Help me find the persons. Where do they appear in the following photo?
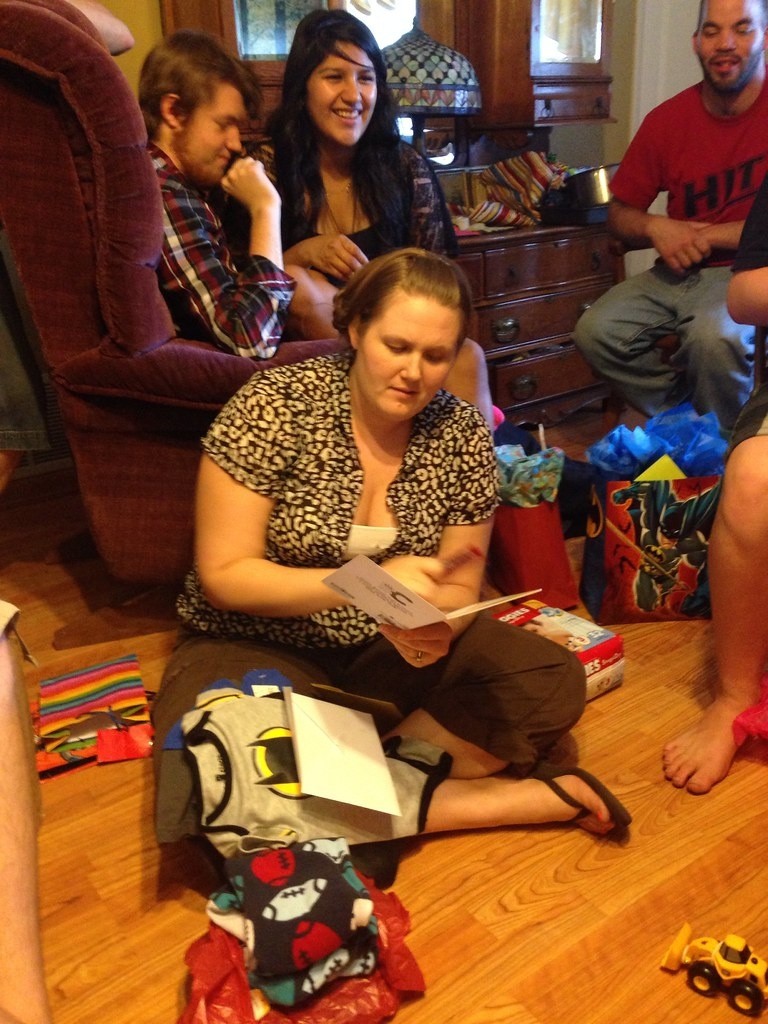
[150,246,632,879]
[661,168,767,795]
[136,6,507,445]
[570,0,768,433]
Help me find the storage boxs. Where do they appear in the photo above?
[492,598,627,700]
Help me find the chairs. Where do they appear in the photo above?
[0,0,349,585]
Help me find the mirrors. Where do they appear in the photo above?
[539,0,604,64]
[234,0,421,63]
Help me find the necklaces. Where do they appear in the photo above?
[325,194,357,235]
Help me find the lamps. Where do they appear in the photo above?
[380,13,483,158]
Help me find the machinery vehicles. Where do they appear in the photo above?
[659,921,768,1018]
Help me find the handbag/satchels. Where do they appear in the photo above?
[40,655,150,752]
[31,690,157,773]
[579,469,724,627]
[488,495,577,610]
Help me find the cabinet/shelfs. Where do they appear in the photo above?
[457,222,626,429]
[419,0,617,125]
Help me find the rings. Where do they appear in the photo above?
[416,651,423,661]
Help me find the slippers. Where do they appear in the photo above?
[506,763,632,832]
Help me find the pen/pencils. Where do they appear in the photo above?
[438,541,481,574]
